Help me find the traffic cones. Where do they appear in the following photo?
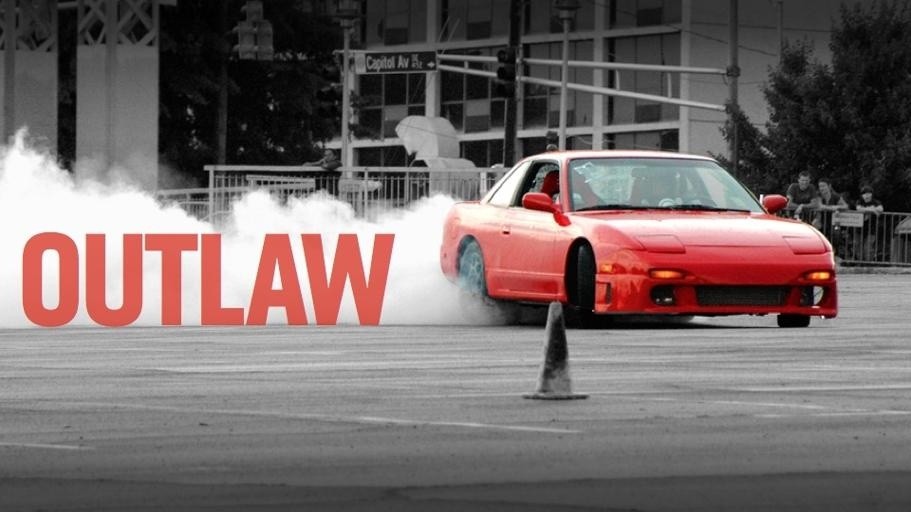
[515,294,594,401]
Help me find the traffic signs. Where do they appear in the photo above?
[349,49,440,77]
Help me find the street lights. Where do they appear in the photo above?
[554,0,583,151]
[334,0,364,208]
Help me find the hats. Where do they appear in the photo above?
[860,186,873,193]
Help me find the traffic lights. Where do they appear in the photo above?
[494,46,516,99]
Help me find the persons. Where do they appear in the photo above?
[855,185,883,267]
[787,170,817,225]
[642,171,691,208]
[816,177,849,257]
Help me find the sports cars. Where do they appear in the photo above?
[437,146,839,329]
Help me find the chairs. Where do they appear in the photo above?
[541,169,650,209]
